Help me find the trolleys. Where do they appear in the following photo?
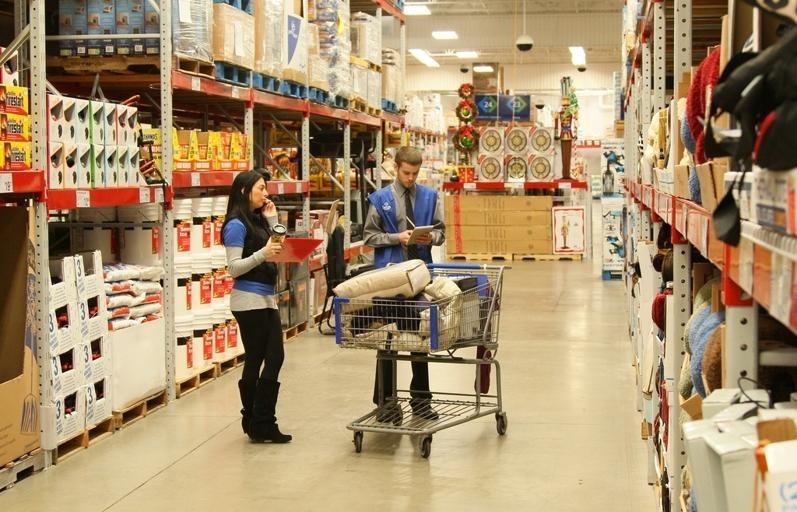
[330,264,512,460]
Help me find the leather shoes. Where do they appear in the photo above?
[377,413,392,423]
[409,401,438,420]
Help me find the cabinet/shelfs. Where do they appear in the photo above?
[0,0,402,487]
[598,1,794,512]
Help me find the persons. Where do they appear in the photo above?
[220,171,292,444]
[363,146,446,422]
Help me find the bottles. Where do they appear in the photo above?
[270,223,287,256]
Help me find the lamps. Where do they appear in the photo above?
[514,0,534,52]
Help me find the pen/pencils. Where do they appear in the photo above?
[404,215,417,228]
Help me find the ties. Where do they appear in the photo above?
[404,189,419,259]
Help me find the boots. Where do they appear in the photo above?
[238,379,292,443]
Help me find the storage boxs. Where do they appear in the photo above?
[442,191,555,258]
